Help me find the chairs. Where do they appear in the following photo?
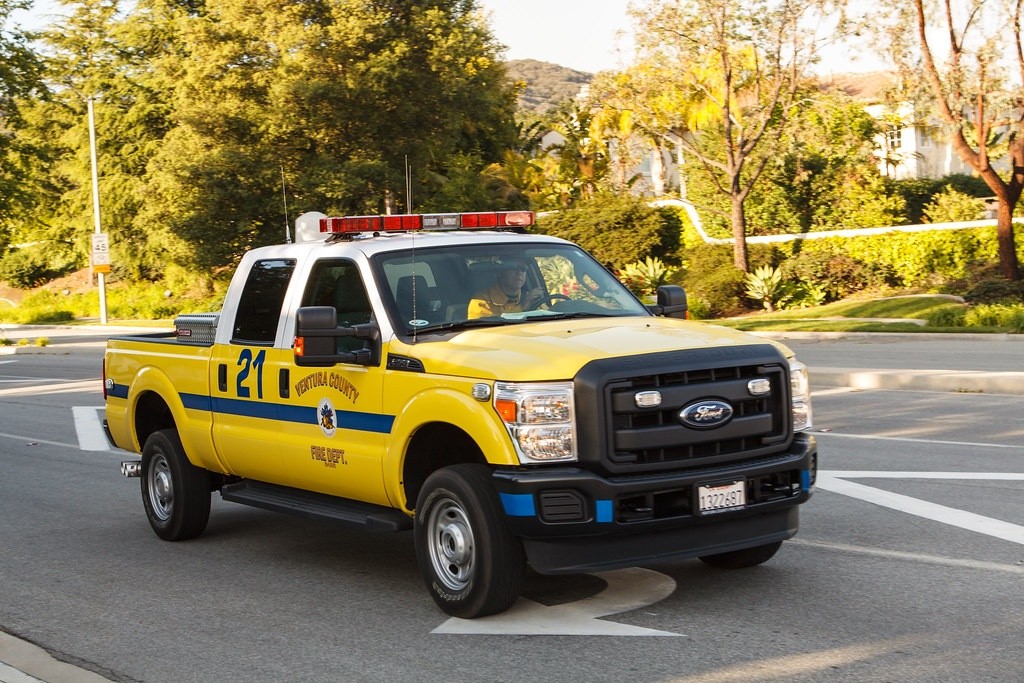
[331,274,438,329]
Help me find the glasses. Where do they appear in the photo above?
[508,263,529,271]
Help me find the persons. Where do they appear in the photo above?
[467,256,549,320]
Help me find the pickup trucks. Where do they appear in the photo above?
[96,209,822,621]
